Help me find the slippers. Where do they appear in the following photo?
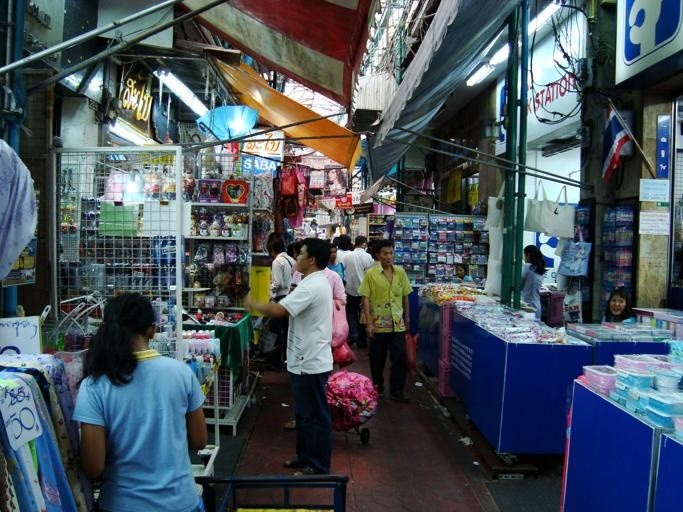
[294,464,329,475]
[285,456,313,468]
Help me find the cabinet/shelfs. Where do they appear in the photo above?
[568,325,673,367]
[181,179,253,310]
[563,379,674,512]
[653,433,683,512]
[239,177,271,344]
[451,300,592,454]
[182,312,254,410]
[367,213,394,243]
[417,294,443,379]
[394,212,428,287]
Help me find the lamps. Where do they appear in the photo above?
[154,70,206,117]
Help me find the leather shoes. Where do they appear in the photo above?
[390,395,410,403]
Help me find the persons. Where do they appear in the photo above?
[327,244,346,281]
[72,292,208,512]
[242,237,333,475]
[335,234,352,287]
[357,239,414,403]
[521,245,549,319]
[342,235,375,347]
[450,264,472,283]
[284,268,347,430]
[266,234,298,361]
[601,289,636,323]
